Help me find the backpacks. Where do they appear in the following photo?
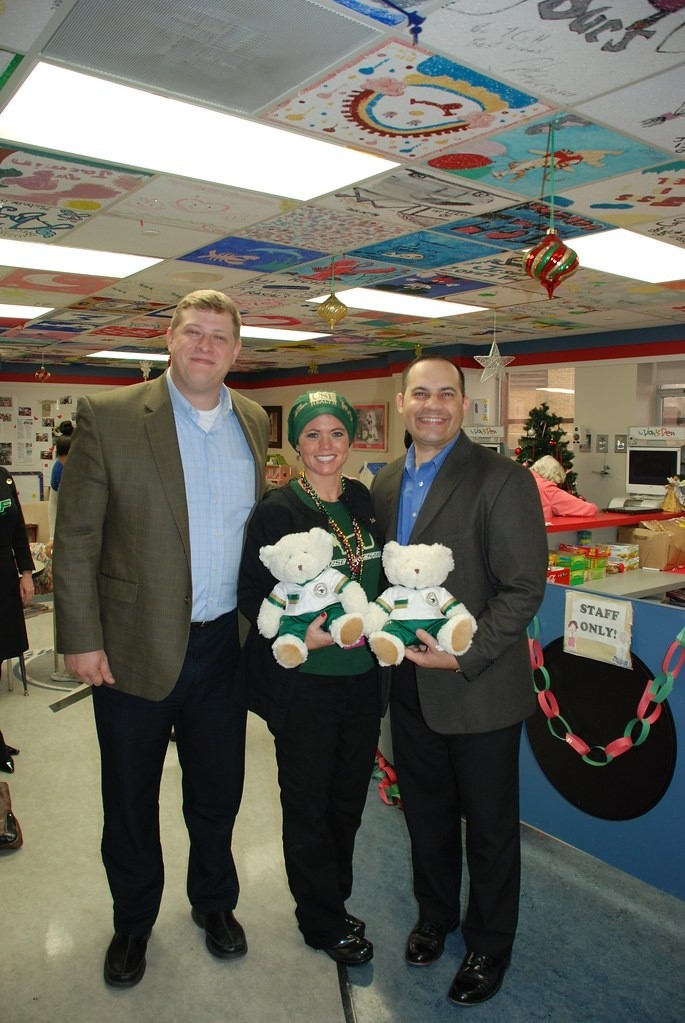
[0,782,22,851]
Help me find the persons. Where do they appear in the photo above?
[50,421,86,685]
[237,389,391,967]
[0,465,38,774]
[52,289,277,987]
[369,354,549,1007]
[529,455,599,519]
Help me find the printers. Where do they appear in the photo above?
[609,497,665,510]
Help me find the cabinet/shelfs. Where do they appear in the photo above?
[265,464,292,488]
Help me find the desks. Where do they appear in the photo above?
[14,558,47,696]
[543,511,685,551]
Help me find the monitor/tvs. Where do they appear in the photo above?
[479,444,500,453]
[626,447,681,495]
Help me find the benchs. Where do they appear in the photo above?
[570,567,685,599]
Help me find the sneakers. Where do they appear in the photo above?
[50,670,83,682]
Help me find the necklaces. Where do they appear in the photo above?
[300,469,364,584]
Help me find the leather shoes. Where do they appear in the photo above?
[449,951,512,1004]
[103,928,154,987]
[0,745,20,774]
[191,906,248,960]
[406,917,460,964]
[322,914,374,966]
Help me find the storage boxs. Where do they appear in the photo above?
[546,517,685,586]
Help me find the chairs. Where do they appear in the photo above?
[8,543,52,693]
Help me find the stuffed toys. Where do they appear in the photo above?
[257,527,373,668]
[365,540,478,667]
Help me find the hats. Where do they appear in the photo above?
[287,390,359,449]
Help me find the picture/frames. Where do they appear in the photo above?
[350,402,388,453]
[261,406,282,449]
